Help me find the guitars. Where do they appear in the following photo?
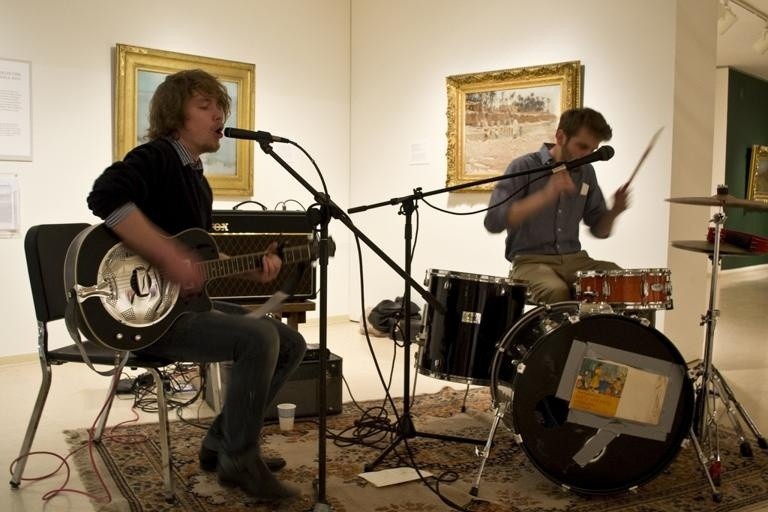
[65,221,334,351]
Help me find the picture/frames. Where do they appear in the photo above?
[443,59,582,195]
[112,42,256,200]
[745,144,768,204]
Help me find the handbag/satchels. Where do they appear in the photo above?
[368,297,421,332]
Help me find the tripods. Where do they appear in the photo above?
[347,164,555,470]
[689,211,768,502]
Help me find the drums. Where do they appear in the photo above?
[415,269,531,387]
[492,300,695,496]
[576,269,673,310]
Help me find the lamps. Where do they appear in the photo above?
[717,1,768,56]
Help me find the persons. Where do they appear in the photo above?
[478,117,522,139]
[85,65,310,501]
[477,101,630,311]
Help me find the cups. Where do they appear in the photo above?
[277,403,297,433]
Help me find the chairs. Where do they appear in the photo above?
[8,221,222,503]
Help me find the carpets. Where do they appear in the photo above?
[60,386,766,512]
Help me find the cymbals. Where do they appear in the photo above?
[672,241,765,255]
[664,196,766,210]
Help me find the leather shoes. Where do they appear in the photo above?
[217,467,300,499]
[199,444,286,472]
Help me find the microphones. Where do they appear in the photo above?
[224,127,289,143]
[256,131,448,512]
[552,145,614,174]
